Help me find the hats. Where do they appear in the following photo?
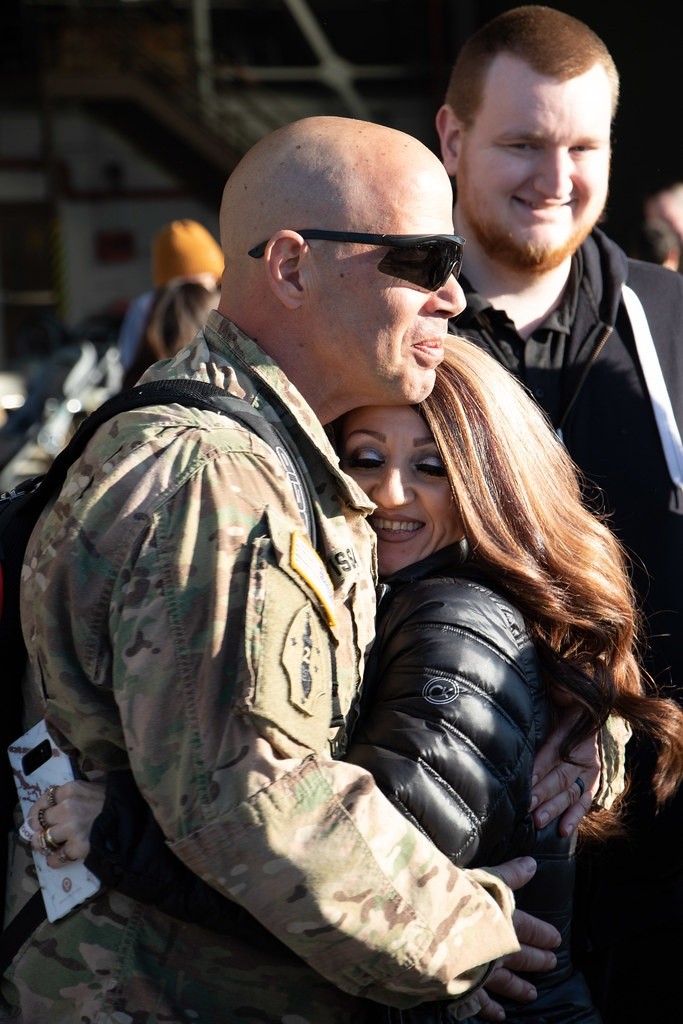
[150,218,226,287]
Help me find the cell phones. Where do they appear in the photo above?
[8,720,105,924]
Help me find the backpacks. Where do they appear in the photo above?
[0,379,319,973]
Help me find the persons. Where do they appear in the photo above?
[0,4,683,1024]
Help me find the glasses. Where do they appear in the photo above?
[248,228,466,292]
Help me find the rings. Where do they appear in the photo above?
[19,817,35,840]
[38,809,48,828]
[47,785,58,805]
[60,847,78,863]
[576,778,585,797]
[38,827,59,854]
